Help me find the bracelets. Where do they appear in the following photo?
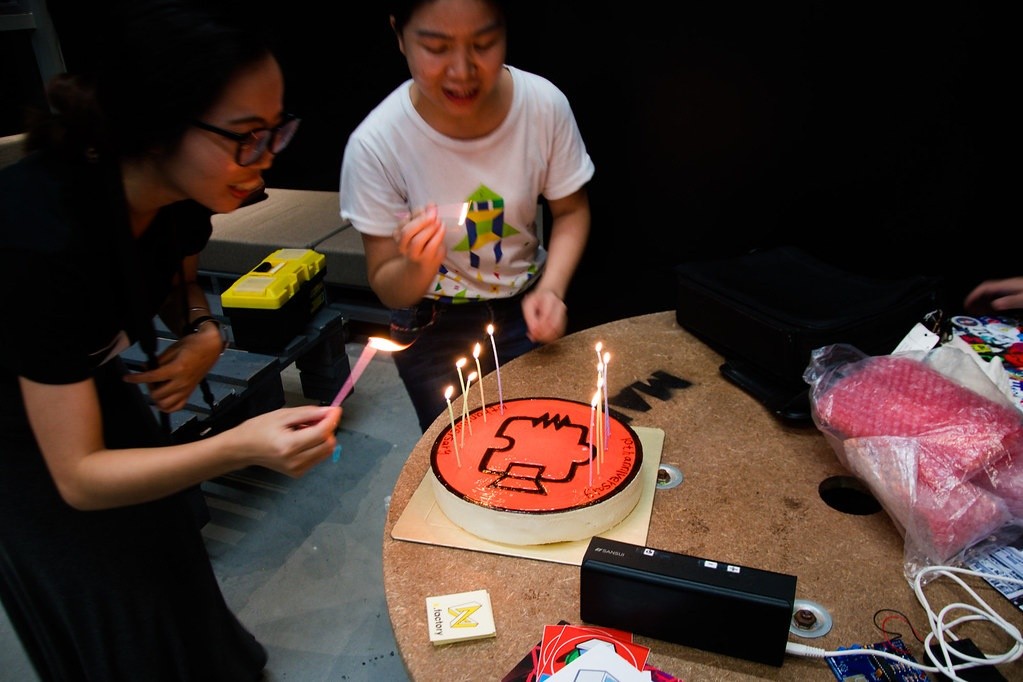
[188,305,211,315]
[191,315,230,356]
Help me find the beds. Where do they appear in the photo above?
[198,187,393,326]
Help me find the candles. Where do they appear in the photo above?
[444,386,462,469]
[330,336,417,406]
[487,323,503,415]
[473,341,486,422]
[589,341,610,486]
[460,372,477,448]
[456,357,472,436]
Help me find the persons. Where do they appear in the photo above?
[964,273,1023,311]
[0,12,343,681]
[340,2,596,447]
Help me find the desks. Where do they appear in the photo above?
[382,309,1023,682]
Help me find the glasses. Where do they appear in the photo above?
[193,111,301,167]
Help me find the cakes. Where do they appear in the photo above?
[428,396,646,547]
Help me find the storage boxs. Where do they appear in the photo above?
[221,249,327,354]
